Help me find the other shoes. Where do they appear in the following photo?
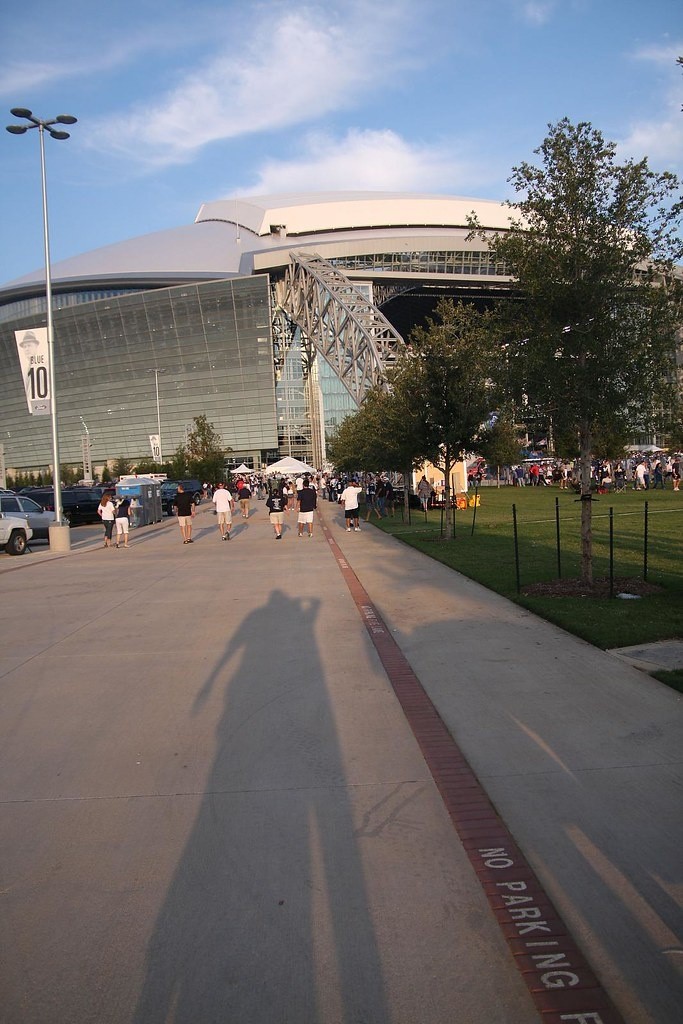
[560,486,564,490]
[564,487,568,489]
[350,523,359,527]
[124,545,129,548]
[117,542,120,548]
[345,528,350,532]
[354,527,361,531]
[653,487,656,489]
[222,532,230,541]
[662,487,666,489]
[288,508,294,510]
[673,486,680,491]
[637,487,641,491]
[308,533,313,537]
[275,535,281,539]
[183,539,192,544]
[299,533,302,537]
[104,542,107,548]
[242,514,248,518]
[645,487,649,490]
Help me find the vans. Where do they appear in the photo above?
[467,457,487,479]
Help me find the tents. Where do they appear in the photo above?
[261,457,318,475]
[230,463,255,474]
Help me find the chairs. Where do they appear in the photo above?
[569,481,629,496]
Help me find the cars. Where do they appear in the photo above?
[0,513,34,556]
[0,495,70,539]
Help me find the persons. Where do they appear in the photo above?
[201,472,321,501]
[496,447,683,493]
[97,493,119,548]
[212,482,235,541]
[265,489,290,539]
[468,469,482,490]
[173,485,195,544]
[114,497,134,548]
[237,483,252,519]
[296,479,318,537]
[316,468,395,532]
[418,476,433,512]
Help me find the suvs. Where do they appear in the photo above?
[0,478,205,524]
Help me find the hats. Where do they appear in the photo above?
[219,483,223,486]
[383,477,389,480]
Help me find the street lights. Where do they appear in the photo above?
[146,366,168,465]
[5,107,78,556]
